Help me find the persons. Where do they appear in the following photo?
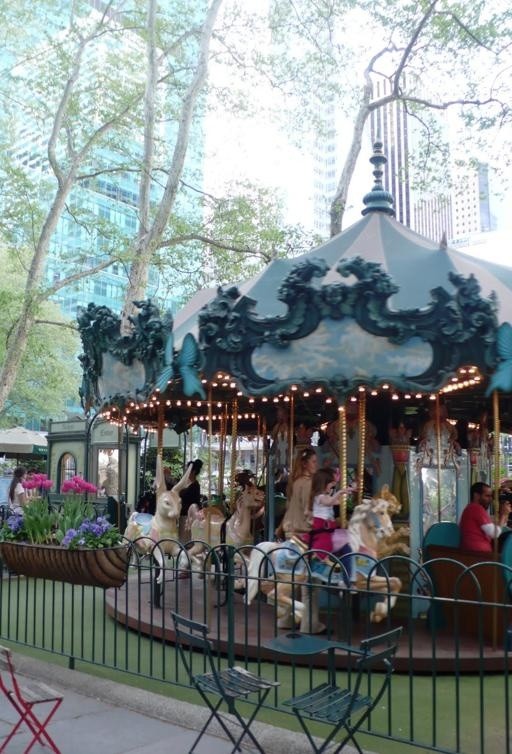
[8,466,28,576]
[458,482,512,553]
[165,459,203,579]
[277,447,327,634]
[301,466,358,595]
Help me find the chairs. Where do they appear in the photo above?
[168,608,404,754]
[1,642,61,753]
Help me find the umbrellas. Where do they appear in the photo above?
[0,426,48,467]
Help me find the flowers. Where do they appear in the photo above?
[1,473,126,548]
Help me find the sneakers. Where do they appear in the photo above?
[175,572,189,579]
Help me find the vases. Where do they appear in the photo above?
[3,542,130,590]
